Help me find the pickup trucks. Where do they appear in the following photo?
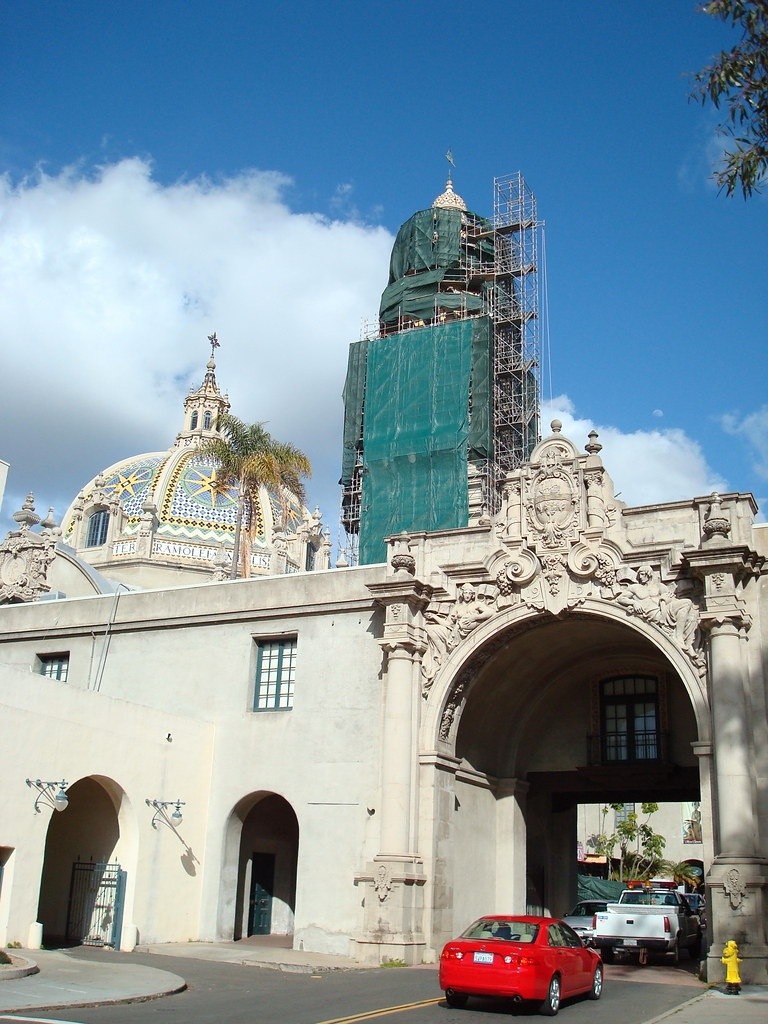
[593,880,707,965]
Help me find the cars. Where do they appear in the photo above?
[439,914,604,1017]
[560,900,625,959]
[680,890,706,925]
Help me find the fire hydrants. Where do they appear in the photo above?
[720,939,744,995]
